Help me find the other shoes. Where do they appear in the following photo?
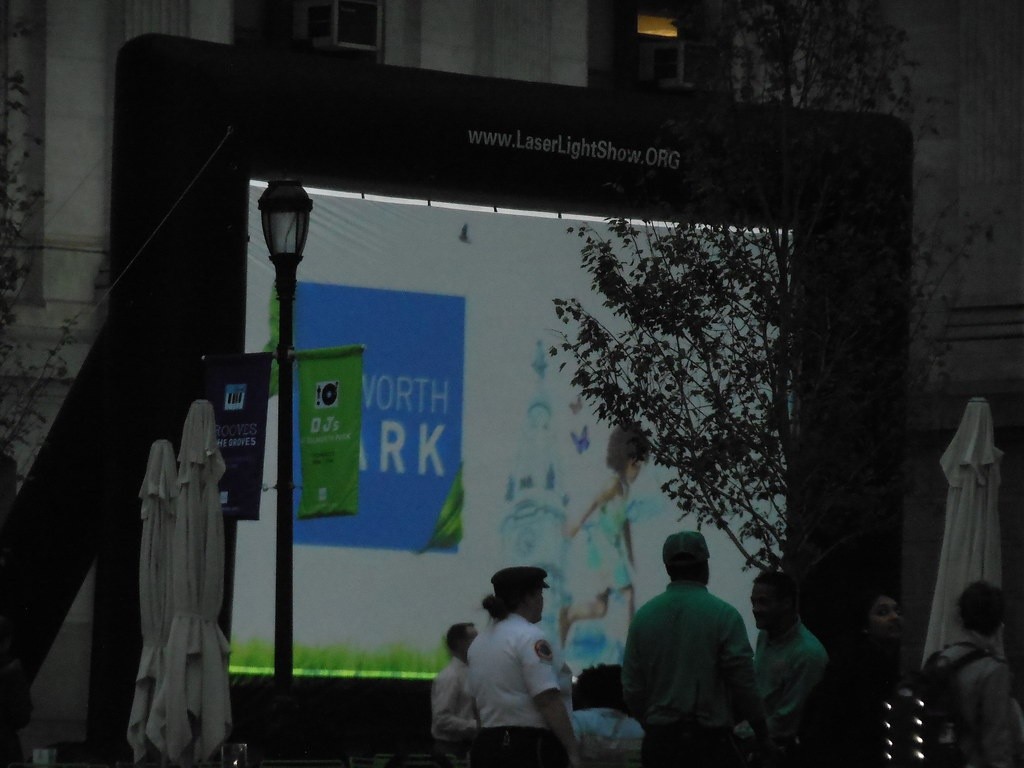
[559,607,572,650]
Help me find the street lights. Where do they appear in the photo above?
[257,174,314,762]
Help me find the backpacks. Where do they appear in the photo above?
[884,642,994,768]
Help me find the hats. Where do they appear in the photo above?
[662,530,710,566]
[491,567,551,596]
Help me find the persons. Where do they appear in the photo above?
[558,420,650,649]
[429,531,1024,768]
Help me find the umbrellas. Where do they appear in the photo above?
[920,396,1006,666]
[126,398,233,768]
